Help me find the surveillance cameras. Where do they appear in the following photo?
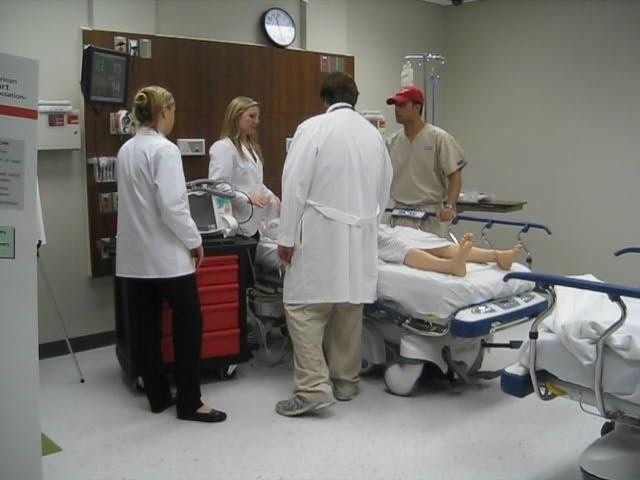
[452,0,462,6]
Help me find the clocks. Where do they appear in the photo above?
[261,6,296,47]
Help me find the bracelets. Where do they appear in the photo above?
[444,204,455,209]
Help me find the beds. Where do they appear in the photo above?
[254,205,553,397]
[501,246,639,479]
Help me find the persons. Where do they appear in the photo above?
[208,97,280,352]
[264,217,524,278]
[113,86,227,423]
[382,83,467,238]
[274,72,393,416]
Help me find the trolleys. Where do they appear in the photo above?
[115,232,257,392]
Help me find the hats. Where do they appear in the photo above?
[387,85,424,105]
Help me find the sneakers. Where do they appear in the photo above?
[151,392,226,422]
[276,386,359,416]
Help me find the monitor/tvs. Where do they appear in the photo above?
[80,45,130,107]
